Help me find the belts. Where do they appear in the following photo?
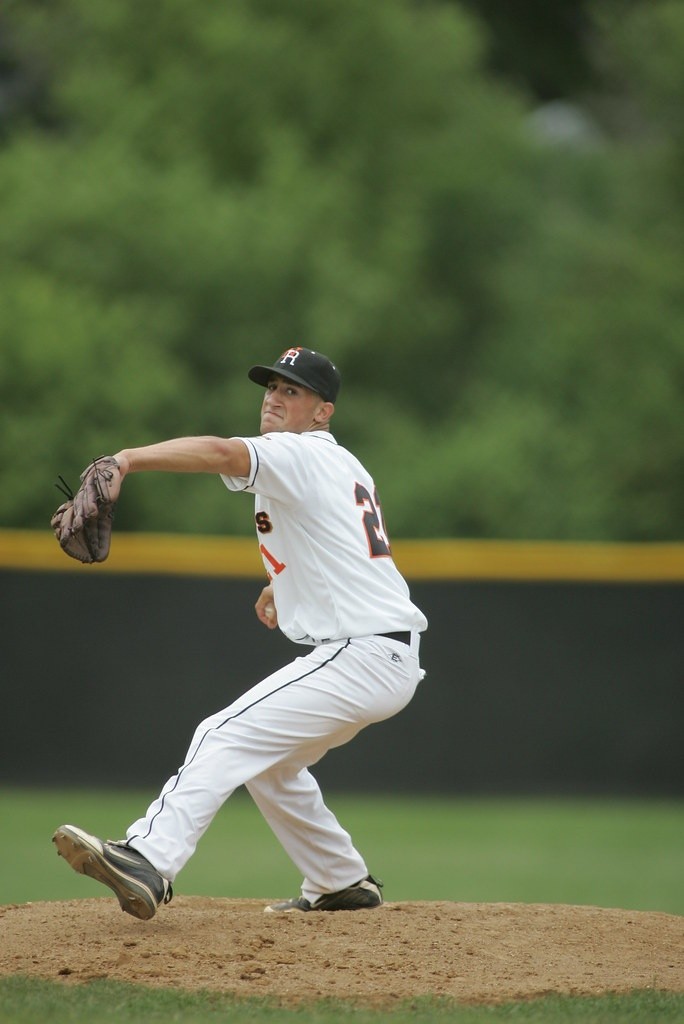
[377,631,414,645]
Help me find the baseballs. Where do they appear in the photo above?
[265,602,276,620]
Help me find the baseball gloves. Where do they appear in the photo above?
[48,454,122,562]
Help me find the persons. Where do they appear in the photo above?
[50,347,428,916]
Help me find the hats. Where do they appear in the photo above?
[248,347,340,406]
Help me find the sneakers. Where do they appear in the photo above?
[263,875,385,912]
[52,824,174,921]
